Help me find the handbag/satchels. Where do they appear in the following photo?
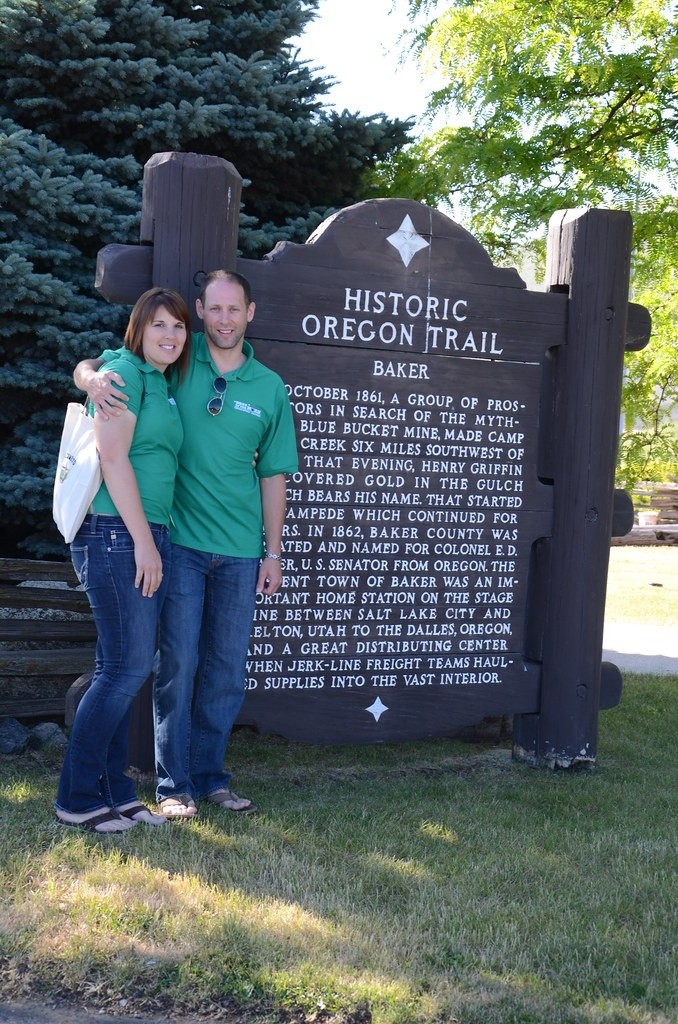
[53,360,148,544]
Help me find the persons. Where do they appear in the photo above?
[73,270,299,818]
[54,290,193,834]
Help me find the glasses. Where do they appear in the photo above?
[207,375,228,416]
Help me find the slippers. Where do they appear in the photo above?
[54,808,136,835]
[158,794,200,818]
[206,790,257,815]
[119,804,171,827]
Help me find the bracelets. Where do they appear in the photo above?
[265,553,282,561]
